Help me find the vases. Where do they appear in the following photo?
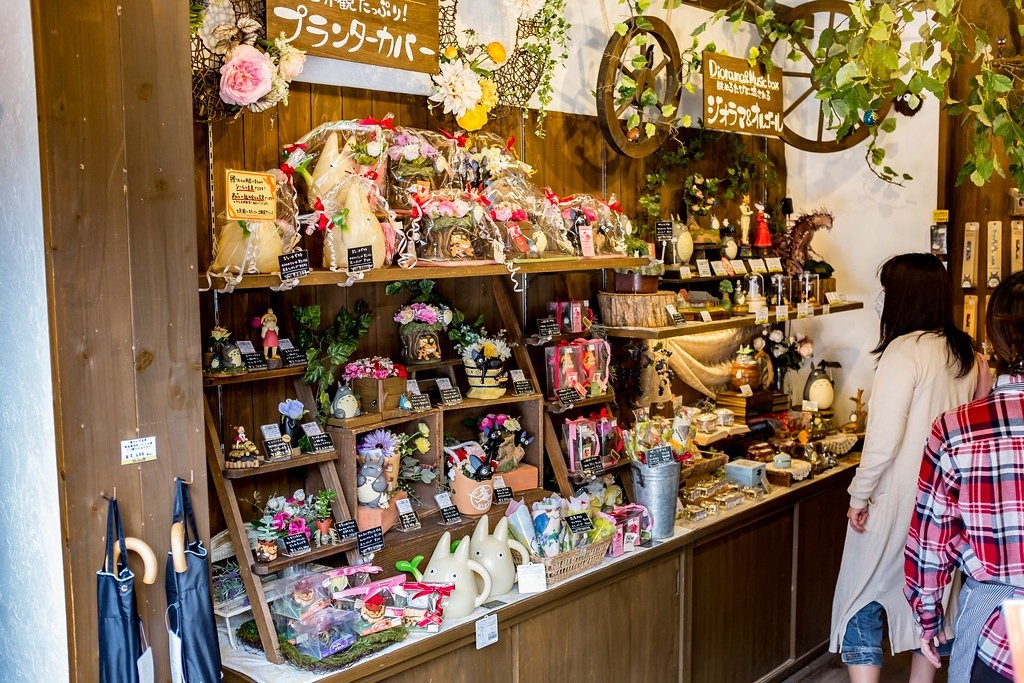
[463,359,510,400]
[479,431,526,472]
[399,320,444,365]
[279,413,304,449]
[257,539,278,562]
[730,363,762,392]
[355,449,401,492]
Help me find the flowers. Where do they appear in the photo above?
[339,353,396,381]
[359,423,433,461]
[278,396,305,426]
[504,491,620,558]
[410,193,475,229]
[214,16,306,116]
[388,131,444,177]
[450,323,513,367]
[575,422,594,439]
[209,558,252,607]
[596,421,615,441]
[753,328,814,370]
[265,489,315,541]
[391,298,449,329]
[424,28,508,132]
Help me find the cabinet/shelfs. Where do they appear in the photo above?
[201,286,375,665]
[199,254,864,339]
[219,451,864,683]
[491,273,636,510]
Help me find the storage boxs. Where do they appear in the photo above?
[543,300,626,474]
[626,507,652,545]
[600,515,634,559]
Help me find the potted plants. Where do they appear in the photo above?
[315,489,337,536]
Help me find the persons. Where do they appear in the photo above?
[754,203,772,248]
[739,193,754,244]
[233,425,248,449]
[261,312,280,361]
[901,271,1024,683]
[827,252,980,683]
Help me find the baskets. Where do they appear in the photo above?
[513,526,615,587]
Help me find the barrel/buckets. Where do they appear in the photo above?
[629,461,682,539]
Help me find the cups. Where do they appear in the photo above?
[453,465,494,515]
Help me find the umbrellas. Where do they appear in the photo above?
[96,537,157,683]
[165,523,222,682]
